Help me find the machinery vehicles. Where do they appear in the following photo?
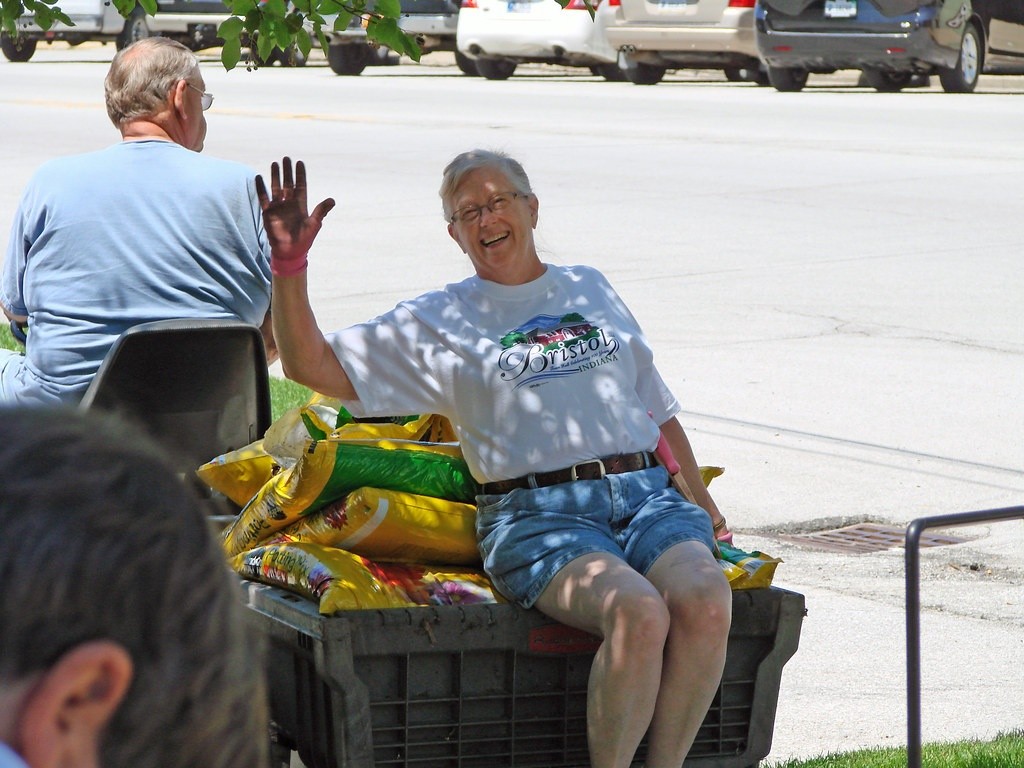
[0,320,809,767]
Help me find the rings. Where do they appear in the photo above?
[280,197,293,201]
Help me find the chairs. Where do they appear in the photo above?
[75,318,274,530]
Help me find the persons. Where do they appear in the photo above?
[256,148,734,768]
[0,398,275,768]
[0,35,285,423]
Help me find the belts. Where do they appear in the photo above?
[476,451,659,494]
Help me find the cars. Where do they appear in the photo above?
[286,1,482,79]
[597,0,770,87]
[0,1,151,65]
[754,0,1024,95]
[144,1,310,69]
[456,0,631,83]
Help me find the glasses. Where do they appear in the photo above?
[173,79,215,111]
[450,192,529,225]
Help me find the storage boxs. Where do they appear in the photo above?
[220,575,811,768]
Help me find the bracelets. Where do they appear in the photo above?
[269,252,309,276]
[714,517,726,531]
[716,532,733,542]
